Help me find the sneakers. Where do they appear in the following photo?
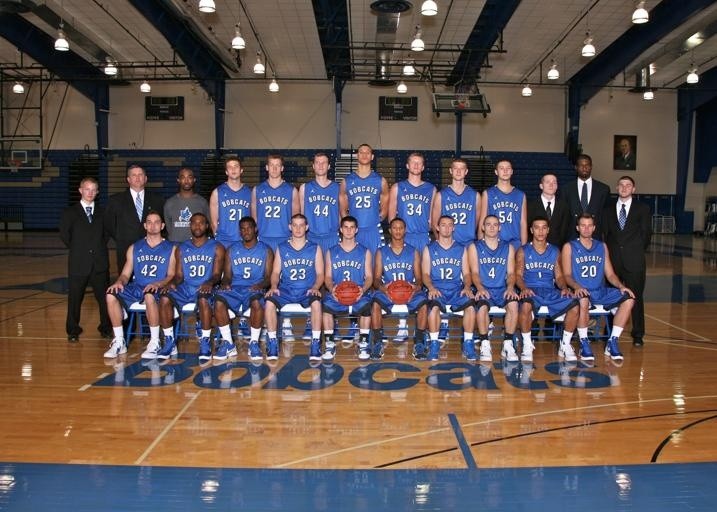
[103,337,127,357]
[604,336,624,360]
[213,361,233,371]
[267,361,278,373]
[141,337,160,358]
[502,358,518,376]
[248,361,262,375]
[341,322,359,343]
[557,339,577,361]
[158,359,174,372]
[198,337,212,360]
[195,320,202,339]
[428,340,440,361]
[393,325,409,341]
[283,342,295,358]
[282,324,295,342]
[519,361,536,376]
[309,338,322,361]
[412,343,428,360]
[577,358,594,368]
[500,339,518,361]
[604,356,623,369]
[322,335,336,359]
[439,322,450,342]
[520,339,535,361]
[265,337,278,360]
[557,359,575,375]
[462,339,480,360]
[358,360,368,381]
[141,359,161,371]
[104,354,126,370]
[310,361,323,382]
[199,360,212,377]
[213,340,238,360]
[301,320,312,340]
[237,319,250,339]
[248,340,263,361]
[334,320,340,340]
[156,335,179,359]
[322,361,335,384]
[578,337,595,360]
[370,342,385,360]
[356,334,370,359]
[394,341,408,358]
[479,340,492,362]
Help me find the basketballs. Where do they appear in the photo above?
[387,280,413,304]
[334,280,360,306]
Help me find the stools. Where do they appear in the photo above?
[215,303,261,354]
[529,305,566,353]
[432,302,474,353]
[377,300,420,351]
[329,305,363,353]
[275,302,324,355]
[579,303,614,353]
[123,300,163,347]
[481,304,518,353]
[174,302,204,351]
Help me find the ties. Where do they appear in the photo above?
[546,202,551,220]
[86,207,93,223]
[619,204,626,230]
[581,183,588,208]
[136,193,142,221]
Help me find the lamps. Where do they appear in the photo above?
[582,38,596,57]
[644,90,655,100]
[547,65,560,80]
[520,84,532,96]
[268,79,280,92]
[198,0,218,14]
[104,57,116,75]
[685,65,699,85]
[403,65,416,76]
[231,23,246,50]
[632,1,650,24]
[139,80,150,93]
[13,81,25,93]
[396,82,408,94]
[420,0,439,18]
[252,52,266,75]
[410,33,425,53]
[55,23,69,51]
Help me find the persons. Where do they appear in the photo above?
[299,153,341,340]
[214,216,275,360]
[480,160,528,343]
[323,216,374,360]
[112,164,160,277]
[388,153,437,342]
[164,168,209,340]
[468,215,520,362]
[266,213,324,360]
[210,157,252,341]
[158,213,225,360]
[561,155,612,341]
[104,211,176,359]
[372,218,428,360]
[339,144,390,344]
[421,216,478,362]
[252,154,300,342]
[617,140,635,168]
[517,218,579,361]
[562,215,635,361]
[431,158,482,346]
[528,173,571,343]
[60,179,115,343]
[605,176,652,347]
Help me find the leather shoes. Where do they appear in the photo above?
[633,337,643,346]
[68,334,78,341]
